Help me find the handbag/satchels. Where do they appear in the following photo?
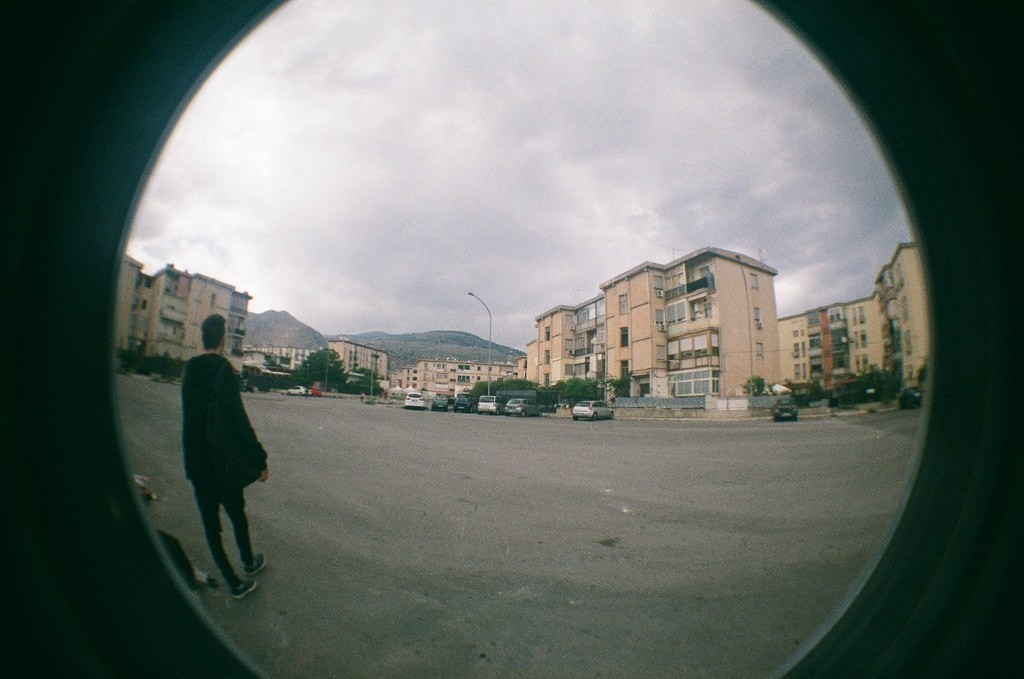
[223,440,266,489]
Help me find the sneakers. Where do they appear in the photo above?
[244,552,265,575]
[230,580,257,599]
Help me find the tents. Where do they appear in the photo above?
[386,385,418,407]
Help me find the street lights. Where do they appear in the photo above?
[468,292,493,396]
[734,254,756,418]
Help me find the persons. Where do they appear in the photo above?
[181,314,269,598]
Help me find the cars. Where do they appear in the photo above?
[504,398,542,417]
[404,392,424,408]
[771,396,798,421]
[900,386,923,410]
[476,395,507,415]
[286,384,313,396]
[572,400,614,421]
[453,397,474,413]
[430,395,450,411]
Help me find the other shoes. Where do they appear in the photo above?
[196,574,221,587]
